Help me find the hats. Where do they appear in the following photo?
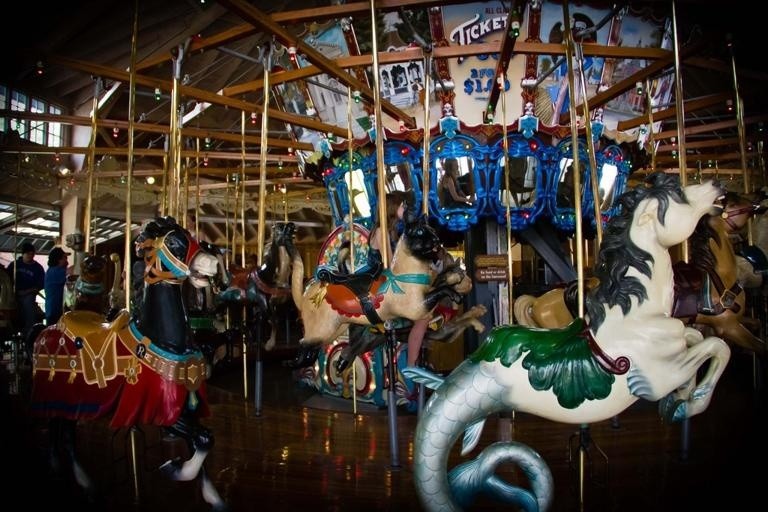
[48,247,72,260]
[21,242,36,254]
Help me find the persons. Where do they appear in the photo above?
[5,243,70,323]
